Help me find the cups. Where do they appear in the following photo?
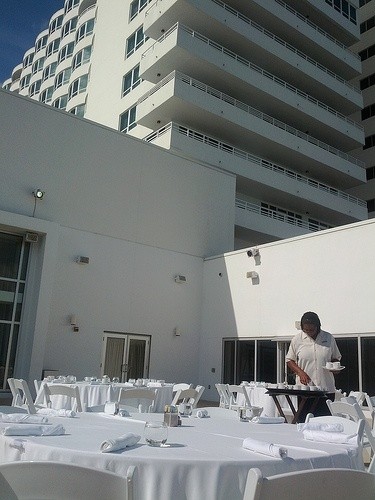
[144,420,168,447]
[242,380,272,387]
[326,361,341,369]
[268,382,322,391]
[84,375,120,384]
[128,378,165,387]
[239,407,249,420]
[47,375,77,383]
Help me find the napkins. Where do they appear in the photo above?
[119,409,129,417]
[297,422,344,432]
[251,417,284,424]
[196,409,208,419]
[101,432,139,452]
[39,407,76,418]
[303,430,359,445]
[2,412,48,424]
[242,437,288,459]
[3,424,64,436]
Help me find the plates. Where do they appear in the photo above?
[321,365,346,371]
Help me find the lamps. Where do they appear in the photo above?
[70,315,76,325]
[26,233,39,242]
[175,274,185,284]
[175,328,180,336]
[75,256,90,265]
[32,187,45,200]
[246,271,258,279]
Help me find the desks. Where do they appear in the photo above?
[40,378,174,412]
[265,387,335,425]
[0,412,365,500]
[236,386,297,417]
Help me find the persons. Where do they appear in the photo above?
[285,312,342,423]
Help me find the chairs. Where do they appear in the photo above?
[243,467,375,499]
[0,461,137,500]
[7,376,375,472]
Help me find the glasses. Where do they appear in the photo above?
[302,326,316,333]
